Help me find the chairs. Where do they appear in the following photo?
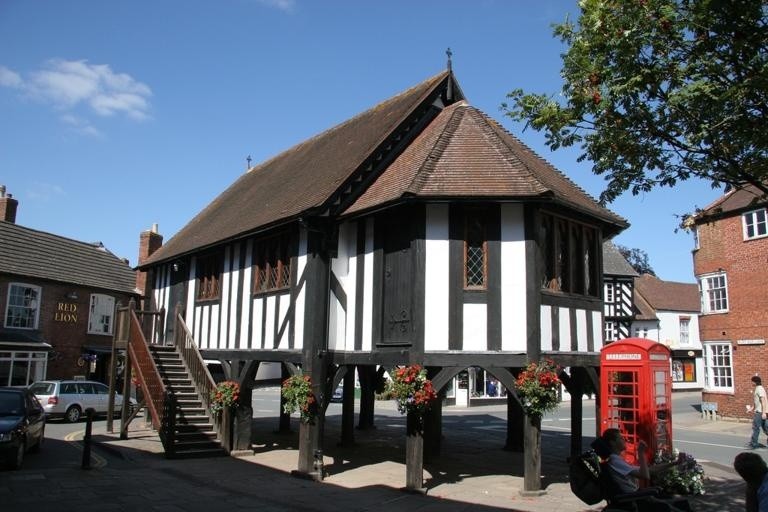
[576,448,680,512]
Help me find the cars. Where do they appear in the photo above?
[0,387,44,471]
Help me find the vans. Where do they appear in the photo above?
[24,378,142,423]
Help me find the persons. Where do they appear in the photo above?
[733,453,768,511]
[550,274,563,291]
[599,428,683,510]
[745,376,768,450]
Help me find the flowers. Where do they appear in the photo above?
[281,375,318,424]
[209,381,241,420]
[374,364,438,420]
[652,451,707,497]
[513,359,564,418]
